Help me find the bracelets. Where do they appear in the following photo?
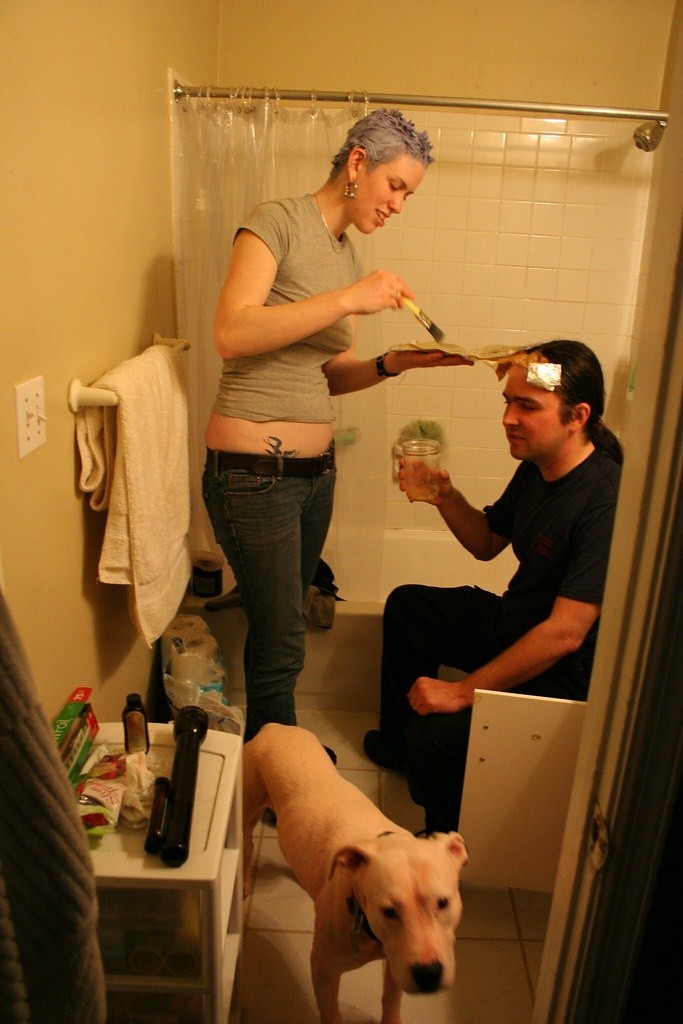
[375,353,402,377]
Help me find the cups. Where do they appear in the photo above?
[193,560,223,598]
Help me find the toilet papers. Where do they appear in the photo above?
[159,614,220,687]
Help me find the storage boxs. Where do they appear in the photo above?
[50,687,100,786]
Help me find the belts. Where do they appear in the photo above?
[203,441,335,478]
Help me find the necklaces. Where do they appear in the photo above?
[312,194,347,255]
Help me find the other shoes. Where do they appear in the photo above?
[365,730,397,771]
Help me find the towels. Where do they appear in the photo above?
[73,348,217,648]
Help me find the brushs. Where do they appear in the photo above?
[403,297,445,344]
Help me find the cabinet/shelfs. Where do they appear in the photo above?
[88,721,246,1024]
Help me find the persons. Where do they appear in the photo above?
[201,108,475,825]
[362,337,625,839]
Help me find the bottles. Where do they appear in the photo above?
[120,693,150,755]
[401,437,439,503]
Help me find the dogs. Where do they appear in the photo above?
[243,723,467,1024]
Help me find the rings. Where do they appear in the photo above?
[392,288,400,298]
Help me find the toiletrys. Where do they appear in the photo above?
[121,692,151,756]
